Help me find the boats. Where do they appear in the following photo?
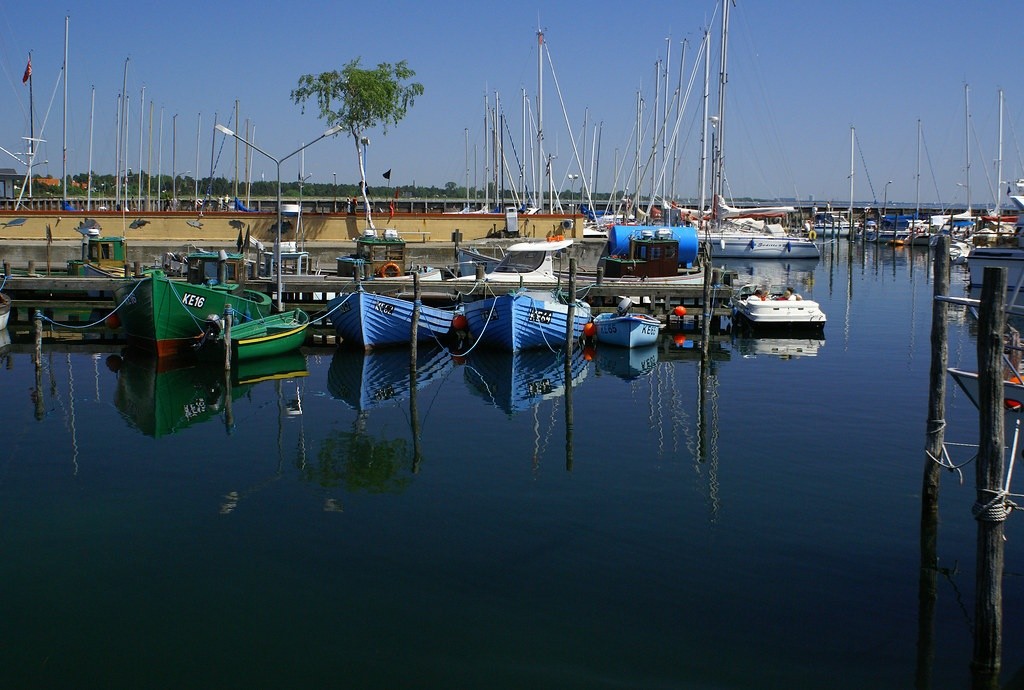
[932,269,1024,461]
[594,297,662,349]
[186,308,309,363]
[110,266,273,358]
[106,337,827,440]
[0,292,12,332]
[730,283,827,332]
[323,263,464,352]
[461,284,593,353]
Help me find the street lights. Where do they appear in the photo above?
[567,174,579,214]
[214,123,339,312]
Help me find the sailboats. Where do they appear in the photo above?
[0,16,1024,338]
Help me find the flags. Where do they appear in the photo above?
[1006,186,1011,196]
[23,56,32,84]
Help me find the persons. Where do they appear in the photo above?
[804,220,810,233]
[131,193,231,211]
[773,214,783,224]
[346,194,358,213]
[684,211,692,226]
[741,286,803,302]
[813,201,830,212]
[964,227,971,237]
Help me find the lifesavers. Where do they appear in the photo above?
[807,230,818,241]
[380,263,401,278]
